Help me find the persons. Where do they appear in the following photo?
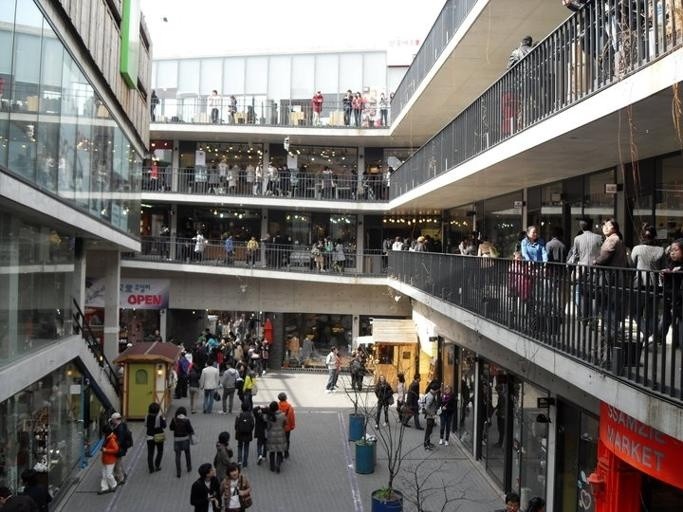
[92,310,314,511]
[459,372,508,449]
[208,90,252,124]
[392,216,683,362]
[313,89,389,127]
[493,492,523,511]
[323,333,454,451]
[525,495,546,510]
[158,226,390,272]
[151,160,392,201]
[504,1,682,133]
[151,90,159,123]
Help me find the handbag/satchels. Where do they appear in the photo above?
[436,405,442,416]
[420,401,426,414]
[190,433,200,445]
[115,447,125,457]
[235,377,244,389]
[396,399,416,418]
[123,430,132,448]
[213,391,220,401]
[239,492,252,509]
[250,351,260,359]
[262,351,269,360]
[251,385,258,395]
[385,395,393,405]
[153,432,165,443]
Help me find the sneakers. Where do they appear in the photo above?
[375,419,437,430]
[424,439,449,449]
[97,476,127,494]
[190,409,231,415]
[173,393,186,399]
[237,454,289,473]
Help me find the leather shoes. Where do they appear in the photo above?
[149,466,160,472]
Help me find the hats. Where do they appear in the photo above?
[107,412,120,422]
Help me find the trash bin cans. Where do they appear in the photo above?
[365,248,382,275]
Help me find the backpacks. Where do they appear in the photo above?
[266,415,286,444]
[237,412,253,433]
